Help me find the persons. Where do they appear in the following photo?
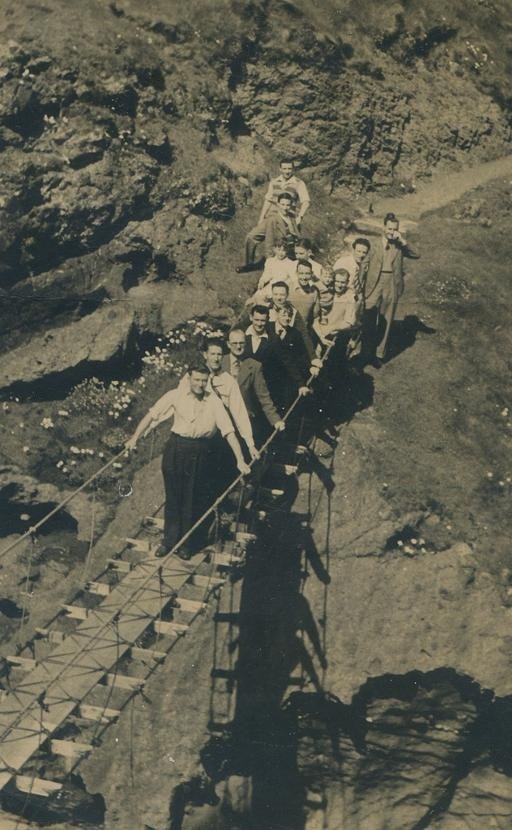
[144,338,261,541]
[236,158,311,273]
[231,238,369,397]
[221,329,285,432]
[125,361,250,558]
[360,217,421,367]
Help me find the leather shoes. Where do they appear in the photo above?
[154,545,169,556]
[236,266,254,272]
[179,546,193,560]
